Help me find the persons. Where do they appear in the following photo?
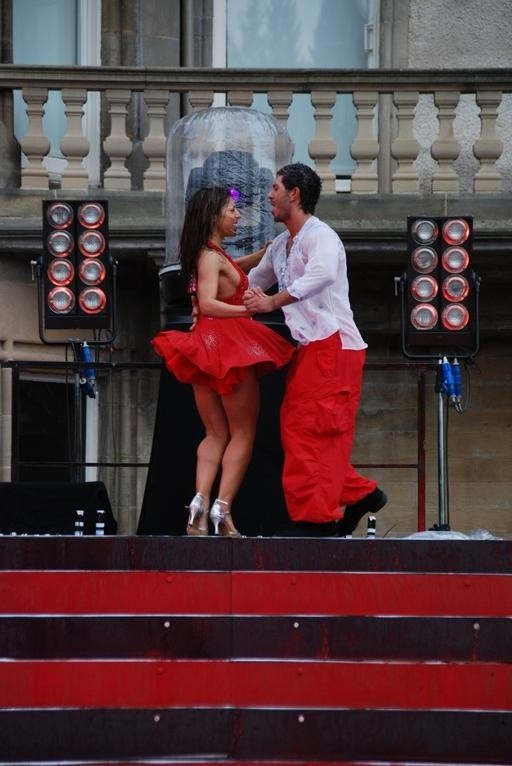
[190,163,387,537]
[150,188,297,543]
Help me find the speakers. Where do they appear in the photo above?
[400,214,481,362]
[40,196,116,330]
[0,480,119,537]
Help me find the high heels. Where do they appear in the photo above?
[184,493,210,535]
[210,499,243,537]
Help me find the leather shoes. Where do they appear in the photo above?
[336,487,388,535]
[275,519,336,536]
